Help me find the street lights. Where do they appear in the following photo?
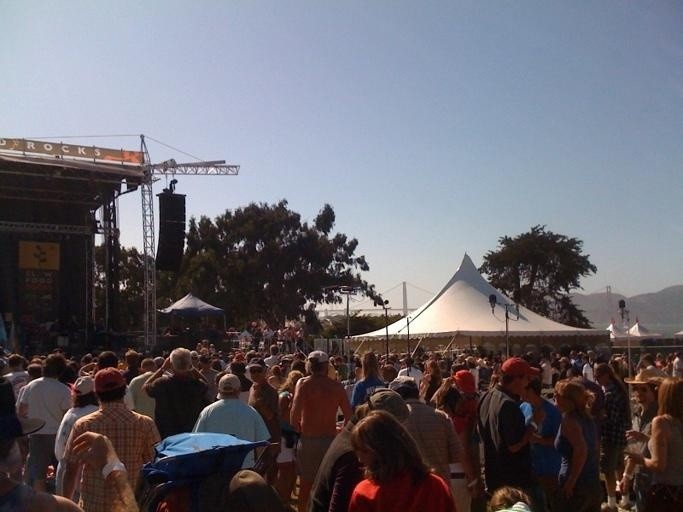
[618,299,631,377]
[489,290,521,358]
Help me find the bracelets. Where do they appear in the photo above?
[531,421,538,430]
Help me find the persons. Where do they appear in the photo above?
[628,378,683,512]
[397,357,422,390]
[124,350,144,383]
[430,370,481,422]
[94,350,125,394]
[264,345,280,367]
[0,377,83,512]
[128,359,168,417]
[200,354,221,401]
[420,361,441,404]
[635,353,666,380]
[154,357,172,376]
[582,354,593,381]
[231,361,252,392]
[18,353,75,493]
[476,358,540,512]
[434,385,476,512]
[140,348,210,441]
[621,372,664,511]
[191,373,271,472]
[554,382,597,512]
[4,354,32,405]
[72,432,140,512]
[306,388,409,511]
[438,360,450,378]
[278,370,303,421]
[389,376,475,491]
[541,354,552,388]
[596,364,633,511]
[194,371,230,424]
[246,359,279,421]
[54,376,102,494]
[15,364,42,399]
[558,356,574,377]
[477,359,491,389]
[673,352,683,377]
[289,350,351,512]
[521,363,561,512]
[354,365,363,381]
[352,351,387,408]
[62,367,162,512]
[269,365,287,387]
[350,410,455,511]
[490,487,531,512]
[335,356,348,380]
[248,321,305,348]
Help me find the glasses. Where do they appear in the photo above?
[192,357,197,360]
[55,349,62,353]
[249,368,263,373]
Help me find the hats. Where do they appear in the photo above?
[368,389,416,424]
[465,356,474,363]
[200,355,212,364]
[558,357,569,362]
[388,376,417,390]
[190,351,198,358]
[8,354,21,366]
[193,468,286,512]
[94,367,124,392]
[244,357,267,369]
[72,376,94,396]
[50,345,64,351]
[624,368,667,384]
[218,374,241,392]
[308,350,329,363]
[502,358,540,377]
[0,377,45,439]
[453,370,476,394]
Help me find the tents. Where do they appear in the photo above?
[157,294,227,332]
[345,253,611,350]
[627,318,662,346]
[606,319,639,346]
